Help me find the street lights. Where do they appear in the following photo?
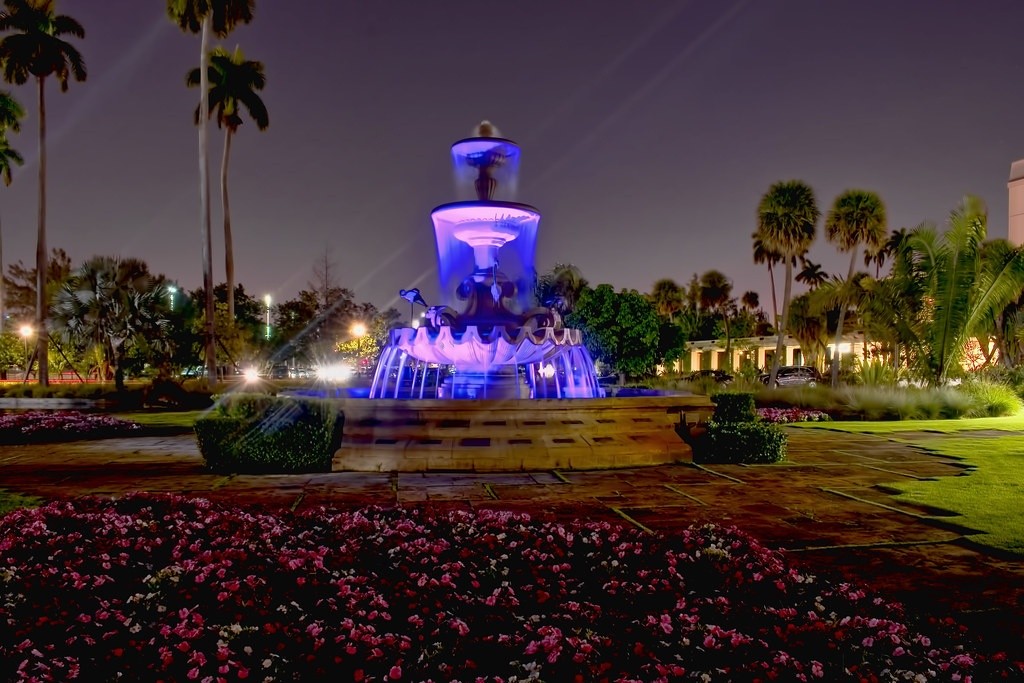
[355,326,363,356]
[168,287,177,311]
[264,294,271,340]
[21,327,31,373]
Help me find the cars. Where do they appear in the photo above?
[675,369,734,383]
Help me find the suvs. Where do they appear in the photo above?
[758,365,820,390]
